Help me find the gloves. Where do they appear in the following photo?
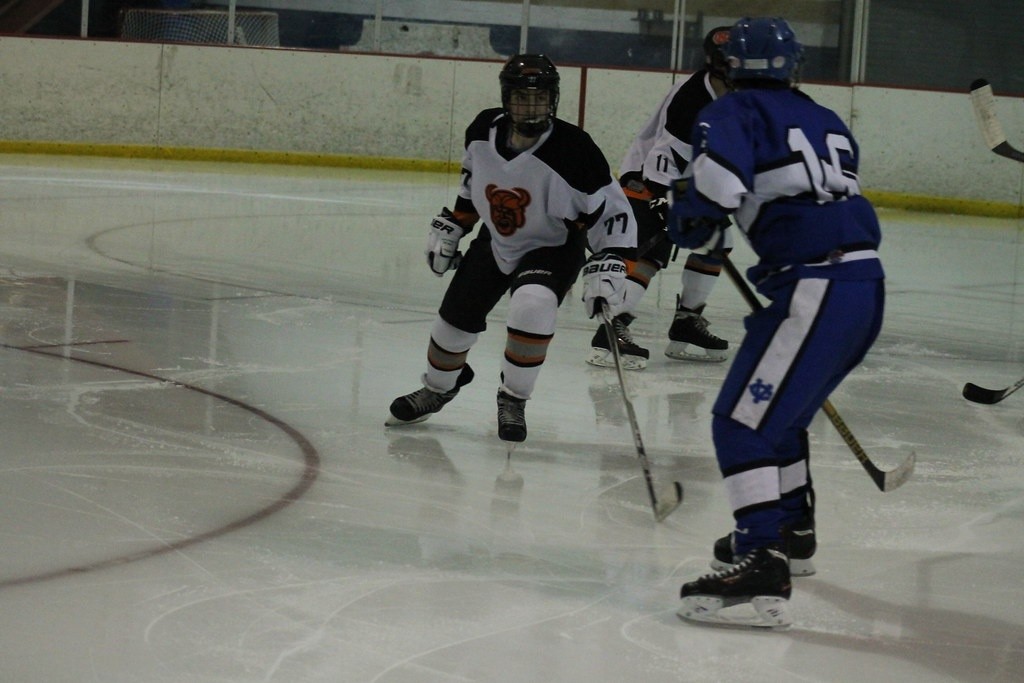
[581,253,627,319]
[424,207,462,277]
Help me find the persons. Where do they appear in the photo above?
[591,16,885,626]
[390,53,637,443]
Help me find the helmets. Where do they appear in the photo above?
[725,16,796,83]
[499,53,561,137]
[703,26,733,80]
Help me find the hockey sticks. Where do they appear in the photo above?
[594,297,687,523]
[960,377,1023,406]
[686,217,918,493]
[965,76,1024,163]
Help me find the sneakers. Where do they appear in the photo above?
[497,371,527,460]
[385,362,474,426]
[586,312,649,370]
[665,294,728,362]
[711,489,816,577]
[676,538,792,626]
